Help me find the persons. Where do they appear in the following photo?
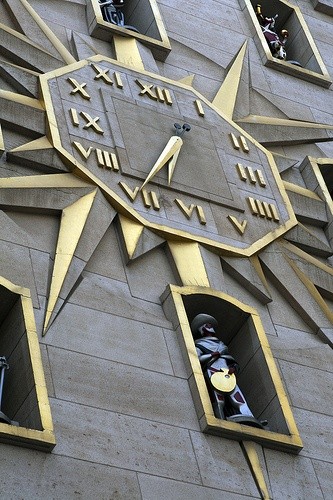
[98,0,127,26]
[256,12,288,61]
[195,322,267,427]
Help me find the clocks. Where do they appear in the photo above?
[35,55,300,257]
